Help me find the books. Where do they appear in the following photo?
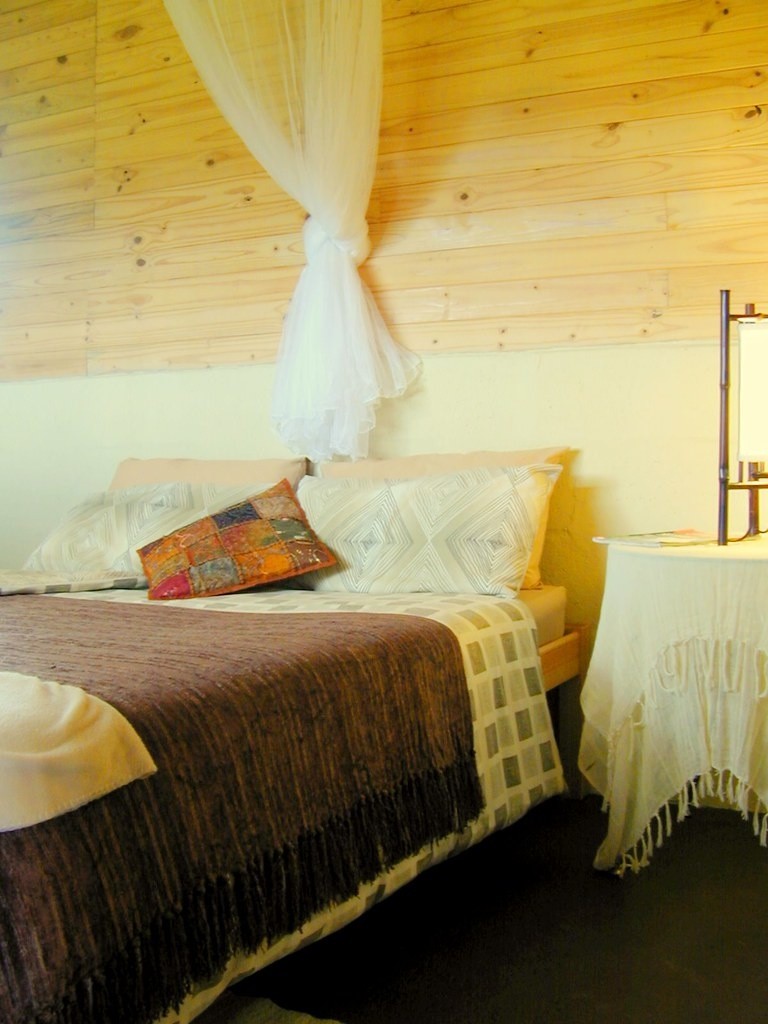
[592,529,740,550]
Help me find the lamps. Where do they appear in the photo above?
[716,288,767,546]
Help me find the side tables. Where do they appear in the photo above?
[604,538,767,813]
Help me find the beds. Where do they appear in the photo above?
[0,570,590,1024]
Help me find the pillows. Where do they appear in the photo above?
[274,463,564,598]
[324,446,567,592]
[135,479,338,601]
[107,460,308,493]
[21,480,294,574]
